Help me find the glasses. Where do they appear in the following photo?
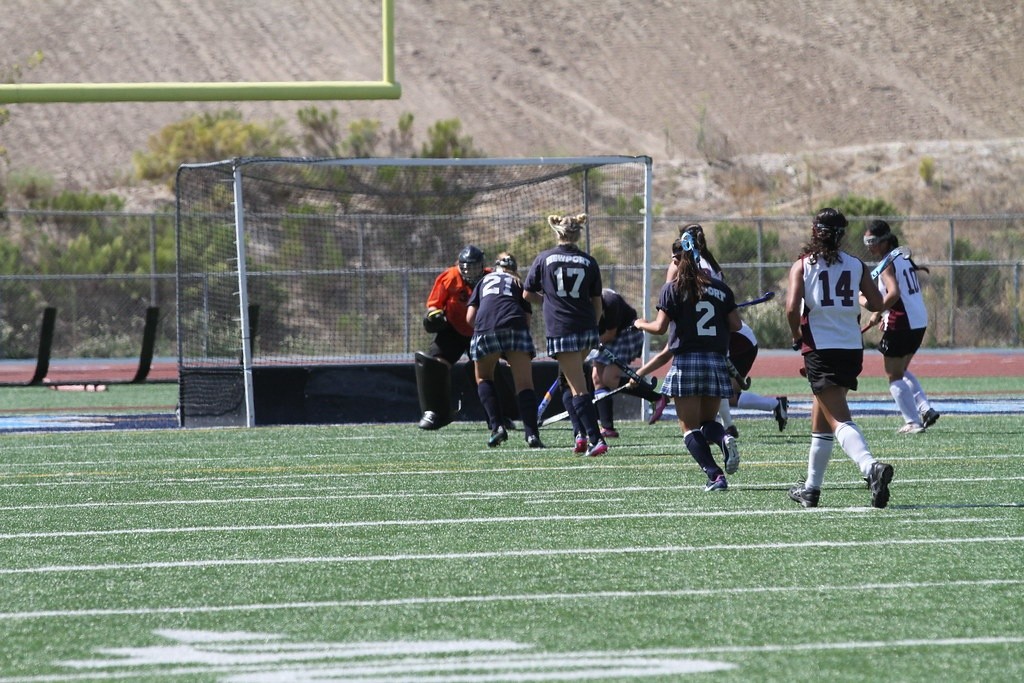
[863,234,883,248]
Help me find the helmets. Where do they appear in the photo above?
[459,244,486,288]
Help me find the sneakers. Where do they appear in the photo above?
[705,475,728,492]
[572,432,587,454]
[648,393,670,424]
[789,474,820,507]
[721,434,741,475]
[487,425,508,448]
[599,428,619,438]
[583,439,607,457]
[527,435,545,448]
[774,396,789,432]
[863,461,893,509]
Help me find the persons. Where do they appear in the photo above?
[786,208,894,508]
[863,219,940,435]
[634,224,790,493]
[416,213,670,456]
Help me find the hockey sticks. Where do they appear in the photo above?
[595,341,657,391]
[537,382,633,428]
[538,376,564,421]
[792,245,914,351]
[798,315,883,378]
[735,290,777,307]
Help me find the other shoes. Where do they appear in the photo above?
[899,421,925,434]
[923,407,940,430]
